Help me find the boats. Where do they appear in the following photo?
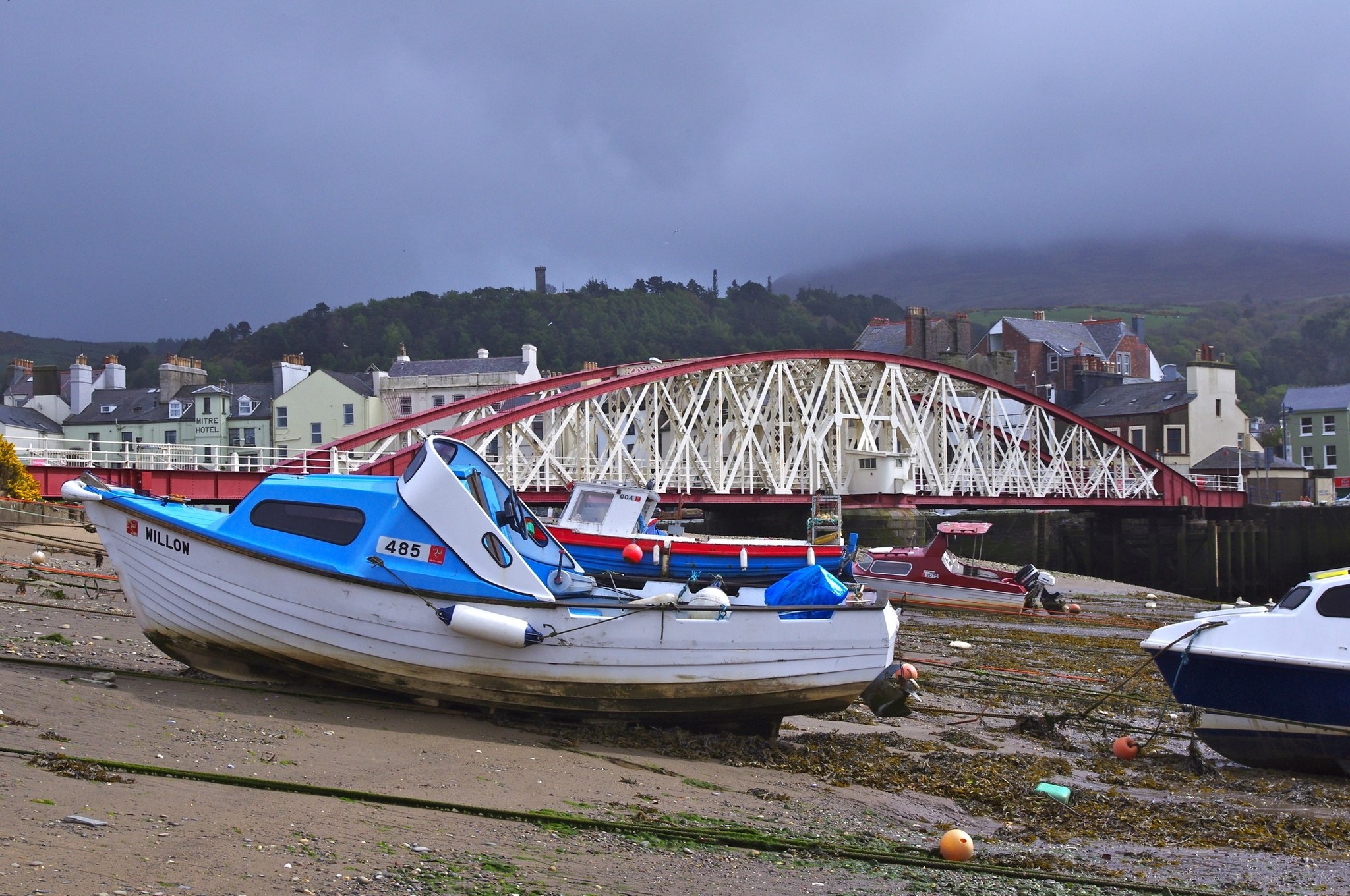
[1140,567,1350,781]
[60,435,1059,736]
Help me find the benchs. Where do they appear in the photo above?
[977,569,1001,582]
